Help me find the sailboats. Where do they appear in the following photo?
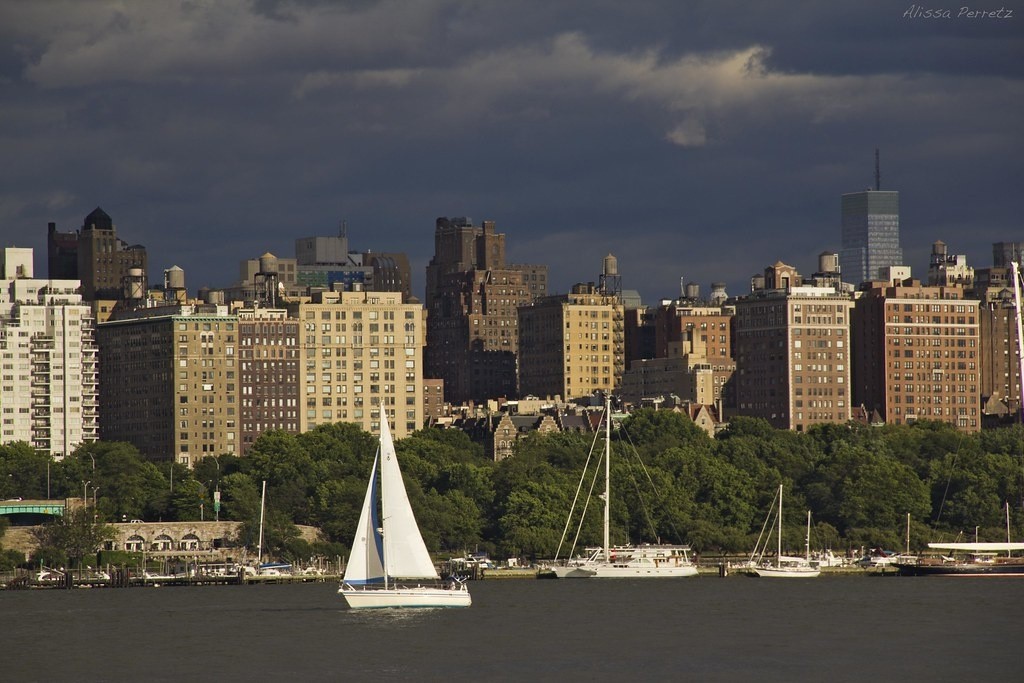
[549,394,699,577]
[752,484,822,577]
[338,399,472,608]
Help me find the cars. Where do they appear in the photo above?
[131,520,144,523]
[917,553,956,566]
[37,573,60,580]
[7,497,22,501]
[41,522,59,527]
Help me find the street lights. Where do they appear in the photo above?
[92,487,100,523]
[976,526,980,552]
[82,480,91,521]
[192,480,213,521]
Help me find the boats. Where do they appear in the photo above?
[450,552,496,568]
[259,564,293,576]
[810,548,848,566]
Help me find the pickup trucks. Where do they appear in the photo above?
[854,556,879,567]
